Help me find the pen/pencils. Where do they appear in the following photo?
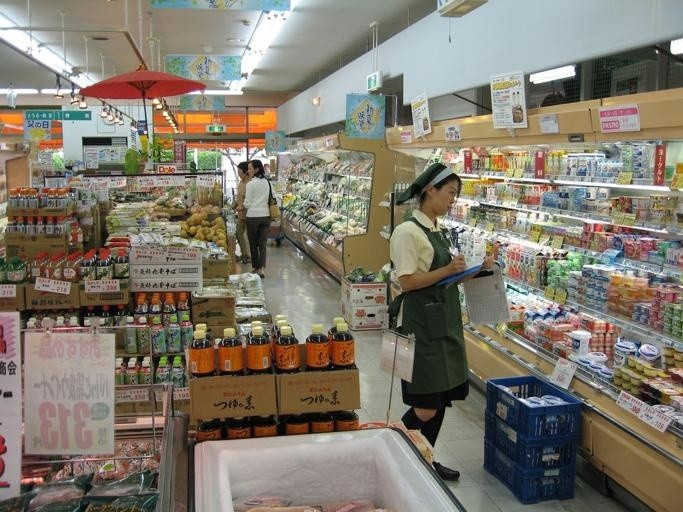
[443,247,455,258]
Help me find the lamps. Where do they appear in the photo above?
[153,96,179,133]
[184,8,289,96]
[0,12,147,134]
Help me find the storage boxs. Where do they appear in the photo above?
[0,210,362,422]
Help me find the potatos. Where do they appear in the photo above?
[179,210,227,249]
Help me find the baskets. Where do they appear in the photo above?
[485,377,584,505]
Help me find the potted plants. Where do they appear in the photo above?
[137,148,148,173]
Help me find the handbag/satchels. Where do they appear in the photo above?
[268,197,281,221]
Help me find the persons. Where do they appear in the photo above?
[231,162,251,264]
[390,162,493,481]
[243,160,273,279]
[262,162,271,177]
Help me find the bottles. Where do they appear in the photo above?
[4,184,76,208]
[0,244,126,283]
[21,293,189,356]
[111,351,192,389]
[2,214,83,249]
[189,407,359,444]
[183,316,355,374]
[521,140,683,428]
[62,159,80,180]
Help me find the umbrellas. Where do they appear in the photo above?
[77,65,207,139]
